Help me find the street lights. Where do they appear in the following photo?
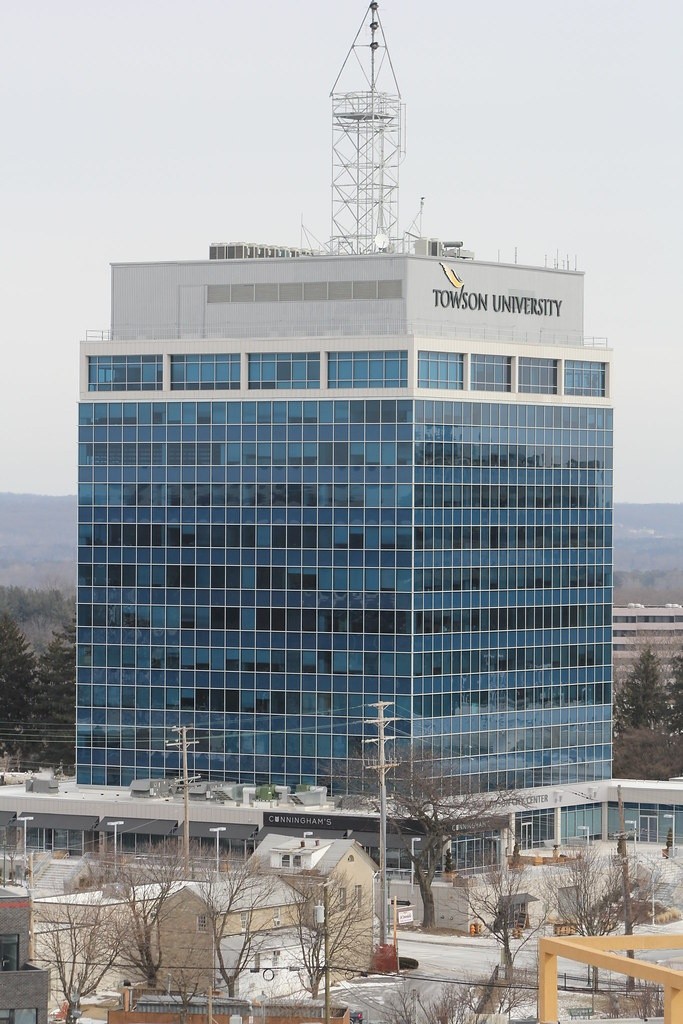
[663,814,675,859]
[624,820,636,875]
[17,817,34,886]
[411,837,422,896]
[209,826,226,882]
[578,825,590,854]
[107,820,124,875]
